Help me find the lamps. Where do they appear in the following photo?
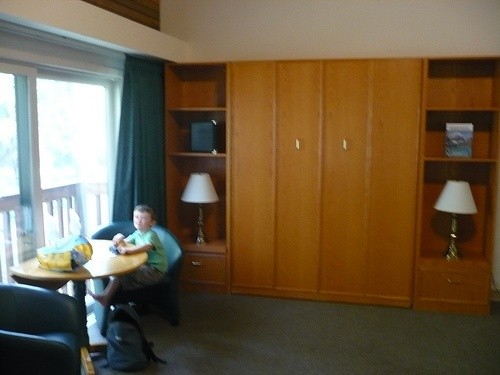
[432,179,478,260]
[180,173,220,249]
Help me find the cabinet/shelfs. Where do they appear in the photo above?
[164,55,500,314]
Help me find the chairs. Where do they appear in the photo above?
[90,222,183,365]
[0,283,84,375]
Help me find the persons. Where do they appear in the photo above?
[87,205,169,306]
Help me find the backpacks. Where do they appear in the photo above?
[100,302,167,371]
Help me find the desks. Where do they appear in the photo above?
[10,239,148,375]
